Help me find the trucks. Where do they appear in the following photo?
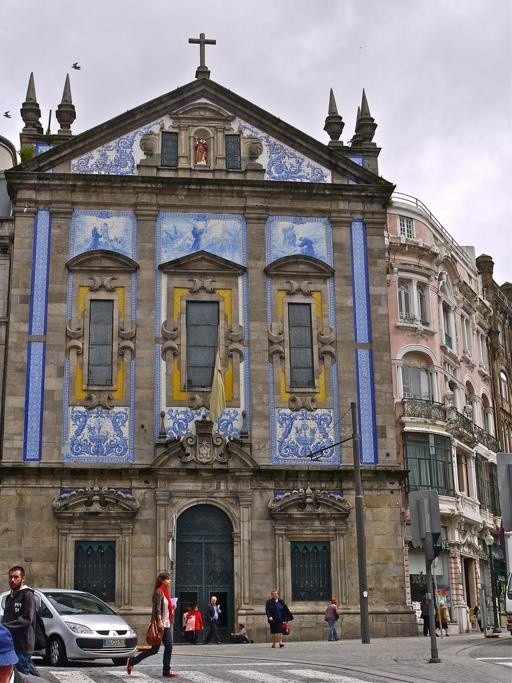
[505,572,512,636]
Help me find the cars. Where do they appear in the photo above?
[1,587,138,665]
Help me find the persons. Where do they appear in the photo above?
[230,623,248,643]
[192,602,203,645]
[1,566,37,683]
[323,596,339,641]
[182,607,193,643]
[125,572,180,678]
[265,590,294,648]
[203,595,224,645]
[0,623,19,683]
[422,594,451,637]
[475,602,485,633]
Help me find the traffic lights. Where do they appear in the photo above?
[430,532,443,559]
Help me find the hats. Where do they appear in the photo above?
[330,598,337,605]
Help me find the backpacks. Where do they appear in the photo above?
[21,590,49,650]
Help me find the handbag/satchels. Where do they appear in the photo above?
[146,619,164,646]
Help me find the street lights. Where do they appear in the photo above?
[484,530,501,633]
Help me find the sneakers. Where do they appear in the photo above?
[424,633,450,637]
[127,657,133,675]
[272,644,284,648]
[162,673,177,679]
